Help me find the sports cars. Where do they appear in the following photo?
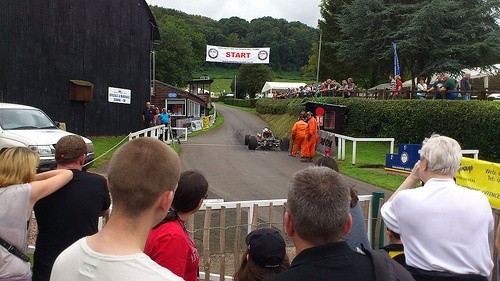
[245,127,289,151]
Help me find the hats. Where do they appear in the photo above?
[55,135,88,160]
[245,228,286,269]
[299,115,304,120]
[306,112,311,114]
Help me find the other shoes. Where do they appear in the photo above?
[310,158,314,161]
[300,158,308,161]
[288,152,295,157]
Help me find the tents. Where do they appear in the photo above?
[261,82,306,98]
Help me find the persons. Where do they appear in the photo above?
[143,101,169,140]
[32,135,111,281]
[50,138,184,281]
[144,170,208,281]
[264,165,414,281]
[388,74,402,99]
[256,127,270,140]
[273,77,356,98]
[289,110,319,161]
[233,227,291,281]
[496,220,500,281]
[315,156,371,252]
[380,134,495,281]
[417,70,472,100]
[0,146,73,281]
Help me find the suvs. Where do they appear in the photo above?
[0,102,95,174]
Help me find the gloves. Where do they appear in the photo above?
[308,134,312,141]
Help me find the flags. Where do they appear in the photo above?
[392,42,400,76]
